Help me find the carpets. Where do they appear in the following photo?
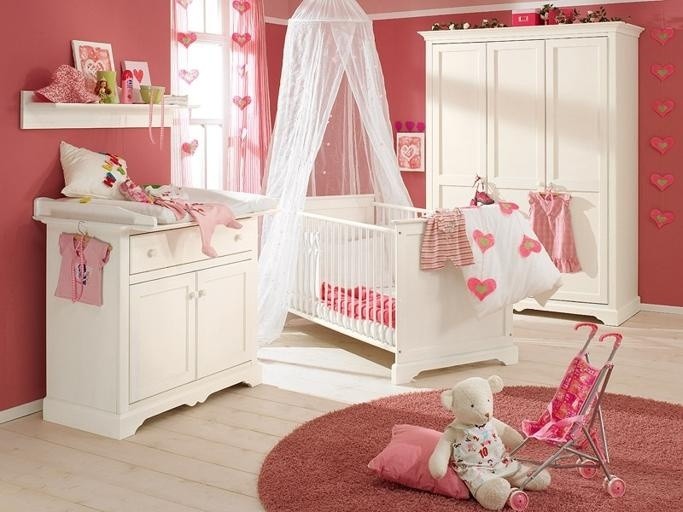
[258,386,683,512]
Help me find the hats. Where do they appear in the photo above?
[34,64,102,102]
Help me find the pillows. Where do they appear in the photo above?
[329,237,393,287]
[368,425,470,499]
[59,141,127,200]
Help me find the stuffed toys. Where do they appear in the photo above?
[428,374,551,511]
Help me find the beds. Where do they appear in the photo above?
[273,194,519,385]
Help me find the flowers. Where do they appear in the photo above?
[554,6,607,24]
[431,18,506,31]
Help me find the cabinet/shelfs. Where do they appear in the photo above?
[32,188,266,441]
[416,21,644,326]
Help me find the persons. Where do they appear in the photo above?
[95,79,113,104]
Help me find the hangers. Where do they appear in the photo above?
[62,221,112,250]
[531,185,571,197]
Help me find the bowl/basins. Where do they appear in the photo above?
[140,84,165,104]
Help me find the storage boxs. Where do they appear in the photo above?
[512,7,541,26]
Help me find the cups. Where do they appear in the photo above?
[96,70,116,103]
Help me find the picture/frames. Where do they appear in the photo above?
[396,132,425,172]
[71,39,115,84]
